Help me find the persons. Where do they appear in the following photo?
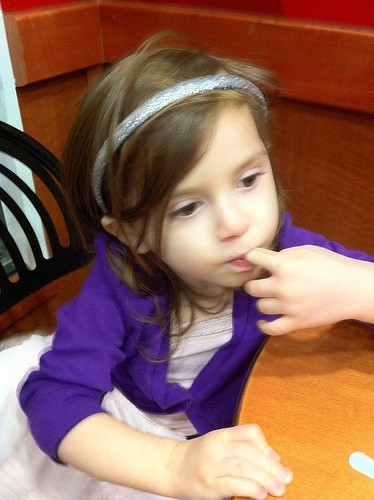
[0,30,374,500]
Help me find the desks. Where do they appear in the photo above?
[235,320,374,500]
